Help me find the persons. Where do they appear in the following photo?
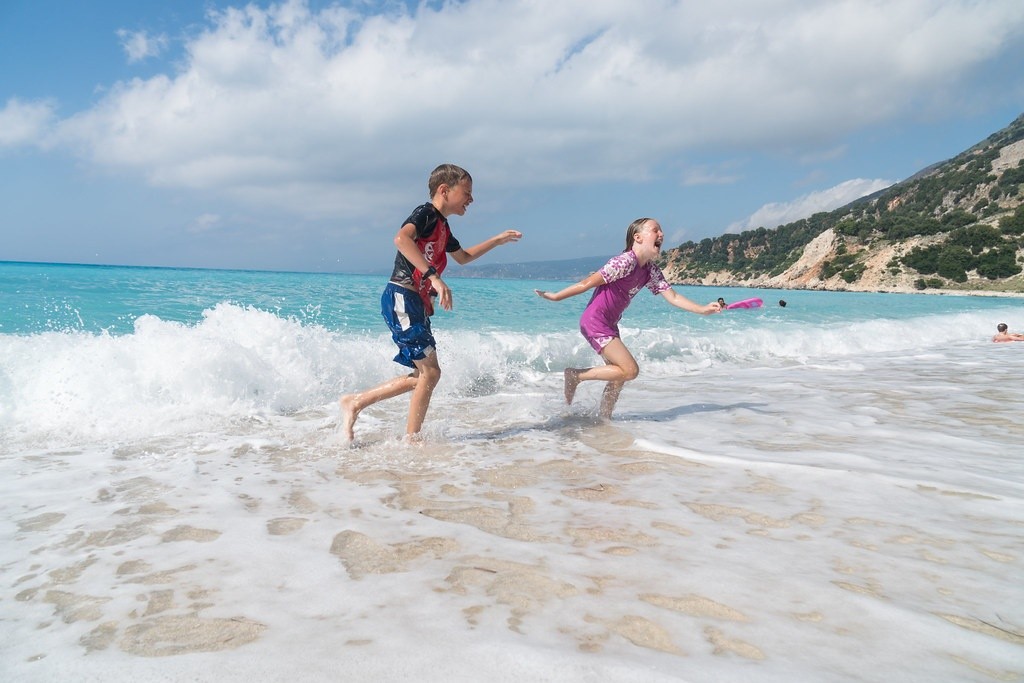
[779,300,786,306]
[342,164,522,441]
[993,323,1024,342]
[535,218,723,420]
[718,298,727,308]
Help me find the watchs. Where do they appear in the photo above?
[422,266,436,279]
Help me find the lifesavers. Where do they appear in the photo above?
[727,297,763,309]
[993,334,1024,343]
